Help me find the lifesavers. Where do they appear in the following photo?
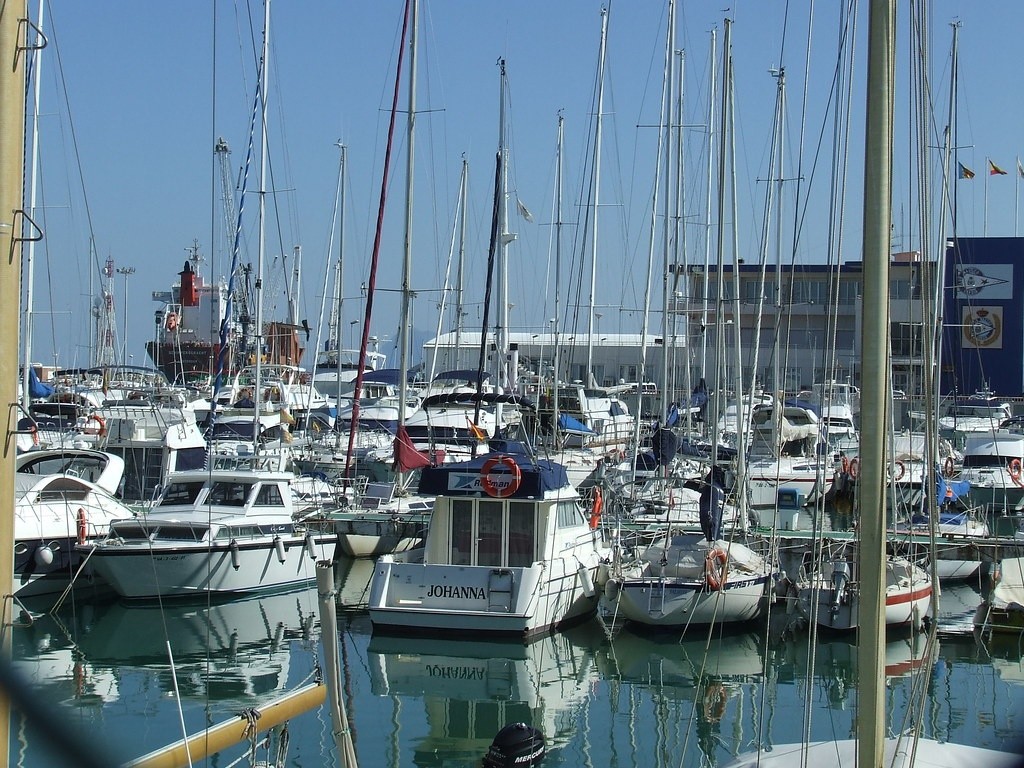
[30,426,38,445]
[77,508,86,545]
[1011,459,1021,479]
[590,496,602,531]
[85,415,104,436]
[888,461,905,480]
[610,449,624,461]
[945,457,953,476]
[701,684,727,723]
[704,547,727,590]
[840,456,860,478]
[480,455,520,496]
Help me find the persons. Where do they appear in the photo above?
[700,466,721,541]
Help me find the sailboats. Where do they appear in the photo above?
[0,1,1024,768]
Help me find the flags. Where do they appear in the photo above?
[1018,160,1024,178]
[958,162,974,180]
[989,159,1008,176]
